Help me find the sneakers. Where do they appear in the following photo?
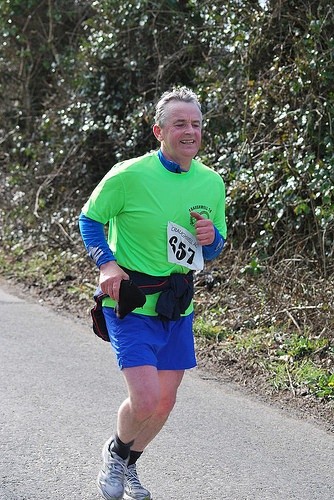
[123,463,151,500]
[97,438,130,500]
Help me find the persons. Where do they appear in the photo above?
[75,84,229,500]
